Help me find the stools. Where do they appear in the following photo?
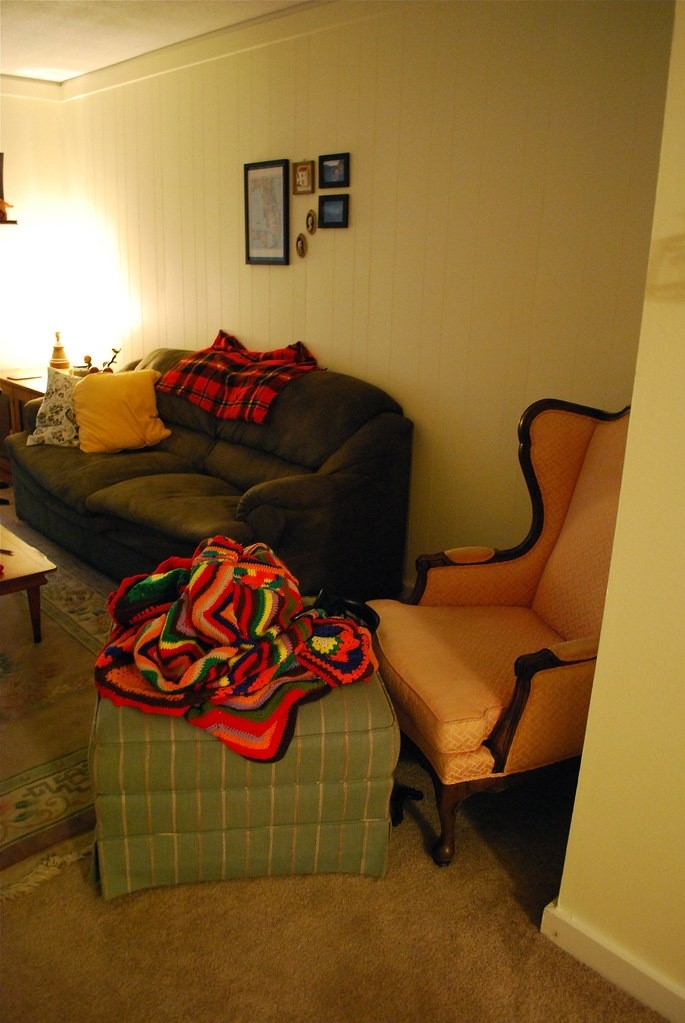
[87,671,400,900]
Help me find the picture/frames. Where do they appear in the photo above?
[318,193,349,229]
[318,152,350,189]
[292,161,314,194]
[244,159,290,266]
[306,210,316,235]
[296,234,307,258]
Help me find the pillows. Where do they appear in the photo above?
[26,366,83,447]
[72,368,172,454]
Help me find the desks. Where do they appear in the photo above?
[0,368,47,434]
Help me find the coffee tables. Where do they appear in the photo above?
[0,524,58,644]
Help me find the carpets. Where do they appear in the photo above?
[0,565,114,874]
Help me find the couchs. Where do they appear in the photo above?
[3,348,415,604]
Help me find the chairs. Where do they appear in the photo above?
[361,398,631,866]
